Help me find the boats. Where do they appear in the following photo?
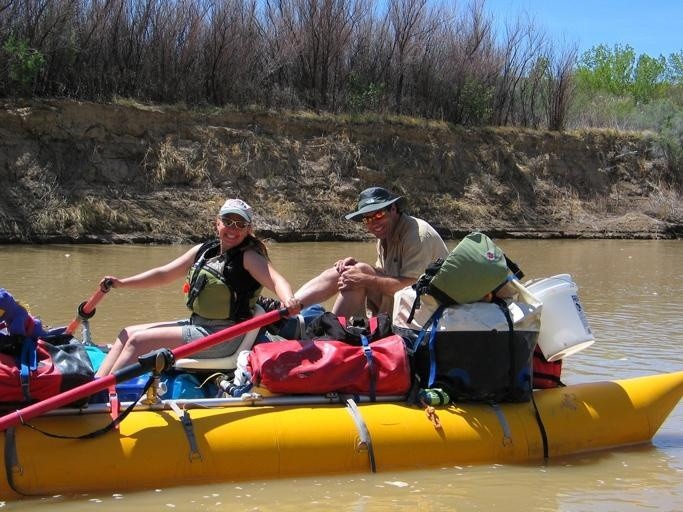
[0,369,682,502]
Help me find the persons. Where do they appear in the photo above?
[253,187,449,323]
[92,196,301,390]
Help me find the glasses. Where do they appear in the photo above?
[219,216,250,229]
[361,210,387,225]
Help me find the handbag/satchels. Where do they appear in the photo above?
[305,311,391,347]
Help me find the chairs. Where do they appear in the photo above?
[173,304,266,370]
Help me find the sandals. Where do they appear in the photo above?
[257,295,280,313]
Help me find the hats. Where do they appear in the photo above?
[219,199,253,223]
[344,187,409,222]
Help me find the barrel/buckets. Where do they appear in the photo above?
[522,272,597,364]
